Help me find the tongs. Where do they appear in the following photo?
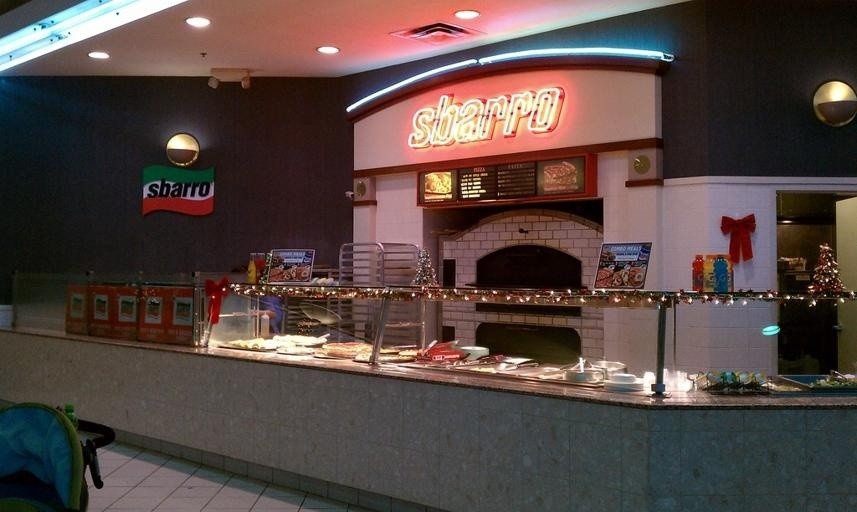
[831,371,847,383]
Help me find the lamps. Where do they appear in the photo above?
[814,81,857,127]
[208,68,250,90]
[167,133,199,166]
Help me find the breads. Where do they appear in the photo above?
[398,349,422,360]
[595,267,629,287]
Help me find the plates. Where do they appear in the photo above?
[604,380,645,392]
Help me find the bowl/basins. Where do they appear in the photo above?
[459,346,489,361]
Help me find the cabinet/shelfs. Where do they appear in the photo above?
[280,268,352,342]
[338,241,425,346]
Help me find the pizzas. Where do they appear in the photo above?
[325,341,374,359]
